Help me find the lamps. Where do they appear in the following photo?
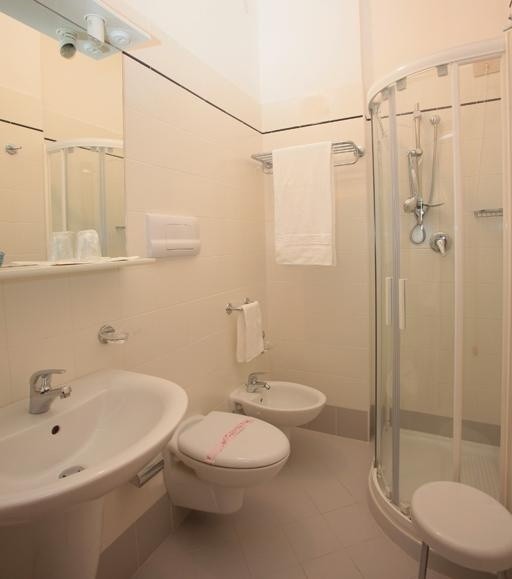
[55,27,78,59]
[84,14,106,46]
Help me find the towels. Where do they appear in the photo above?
[236,300,265,363]
[272,141,337,265]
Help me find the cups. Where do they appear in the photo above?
[51,231,73,264]
[76,229,102,263]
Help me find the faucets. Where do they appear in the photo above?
[29,370,72,415]
[248,371,271,392]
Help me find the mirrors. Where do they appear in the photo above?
[1,0,157,279]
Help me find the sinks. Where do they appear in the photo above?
[229,382,327,426]
[0,370,189,526]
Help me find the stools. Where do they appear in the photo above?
[410,480,512,579]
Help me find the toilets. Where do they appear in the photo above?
[163,411,290,515]
